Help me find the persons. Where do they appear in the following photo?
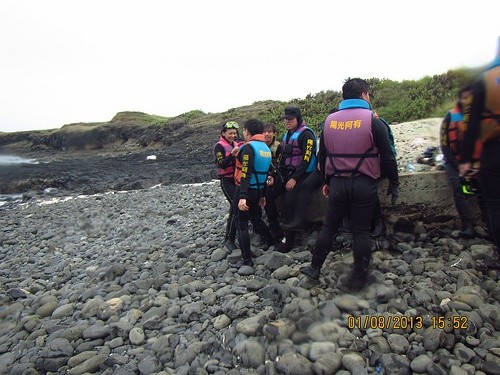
[282,108,351,253]
[458,66,500,274]
[439,87,487,237]
[263,106,317,253]
[372,111,397,237]
[264,124,280,156]
[299,79,399,291]
[228,119,276,269]
[214,120,240,252]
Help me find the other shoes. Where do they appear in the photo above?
[282,220,305,231]
[300,266,320,279]
[229,259,255,268]
[274,242,293,252]
[260,239,273,250]
[225,240,237,251]
[459,227,476,239]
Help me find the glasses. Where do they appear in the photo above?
[226,122,239,128]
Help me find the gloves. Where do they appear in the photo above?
[387,181,399,204]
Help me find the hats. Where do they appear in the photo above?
[282,107,301,119]
